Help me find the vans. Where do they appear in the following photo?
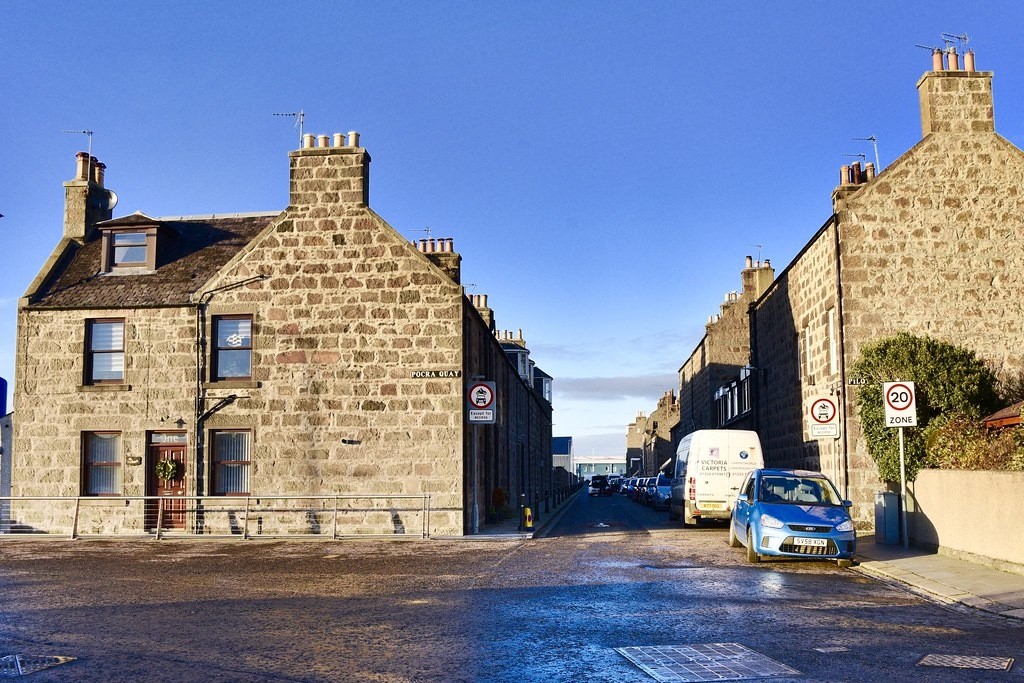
[667,429,765,527]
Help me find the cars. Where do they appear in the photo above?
[588,474,611,496]
[729,468,857,567]
[604,473,657,508]
[651,471,676,511]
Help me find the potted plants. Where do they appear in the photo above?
[487,505,496,524]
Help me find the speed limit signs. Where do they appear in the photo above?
[886,384,913,410]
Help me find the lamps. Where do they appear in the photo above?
[160,413,169,424]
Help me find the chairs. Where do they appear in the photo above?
[764,478,819,502]
